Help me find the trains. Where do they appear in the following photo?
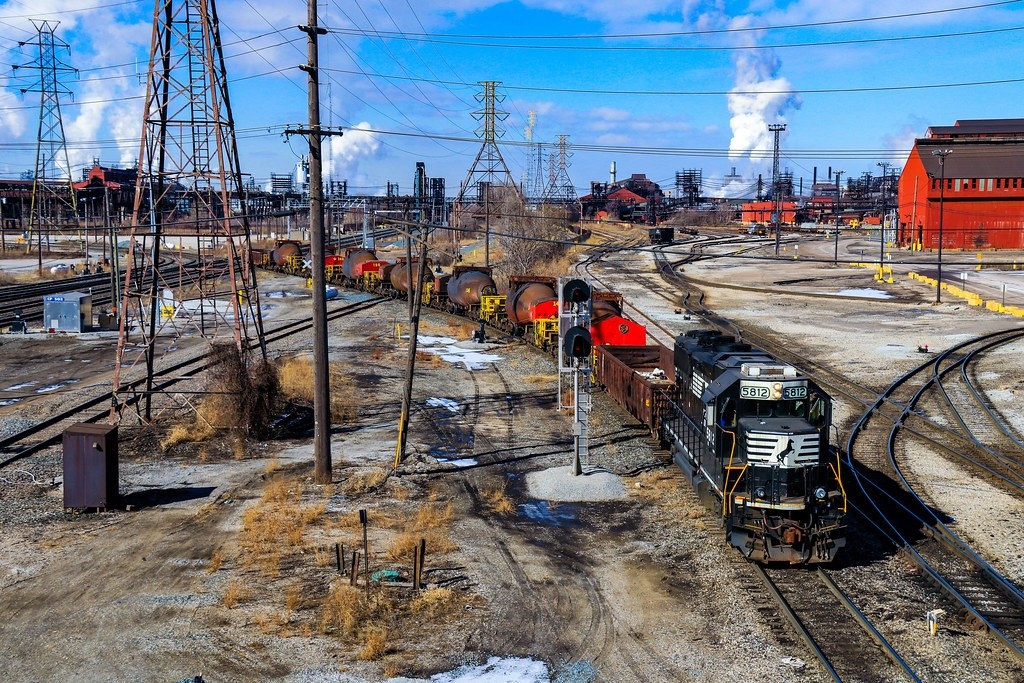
[252,239,848,566]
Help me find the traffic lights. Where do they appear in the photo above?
[559,276,591,372]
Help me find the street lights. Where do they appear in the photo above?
[833,170,847,267]
[932,149,954,305]
[877,162,893,280]
